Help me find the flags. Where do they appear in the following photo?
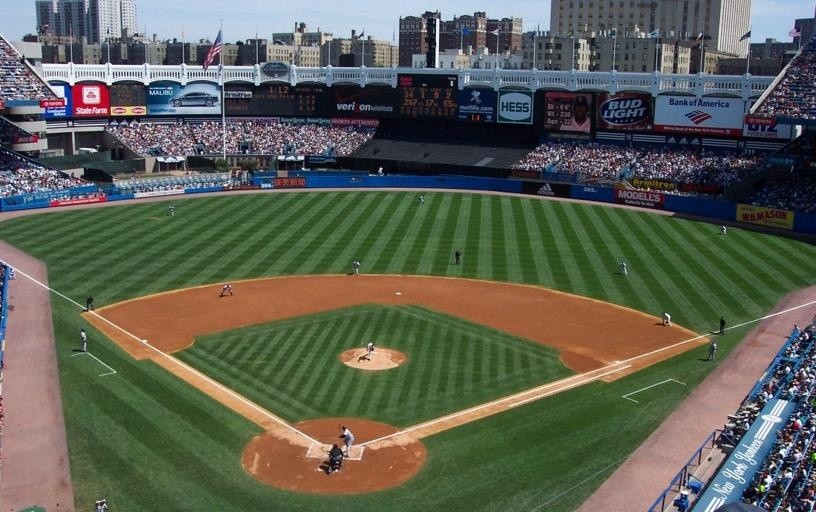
[459,26,704,43]
[790,25,803,37]
[201,28,223,74]
[739,32,752,42]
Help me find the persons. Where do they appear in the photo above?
[325,443,344,475]
[79,327,88,352]
[661,311,672,327]
[417,193,424,206]
[375,165,384,177]
[85,296,96,311]
[618,261,628,276]
[720,224,726,235]
[218,284,233,296]
[94,498,109,512]
[0,114,105,204]
[673,314,816,511]
[0,37,59,103]
[105,118,378,159]
[454,249,462,265]
[337,426,355,459]
[0,261,17,426]
[351,259,360,279]
[167,204,175,216]
[749,36,816,119]
[105,159,275,197]
[364,342,376,360]
